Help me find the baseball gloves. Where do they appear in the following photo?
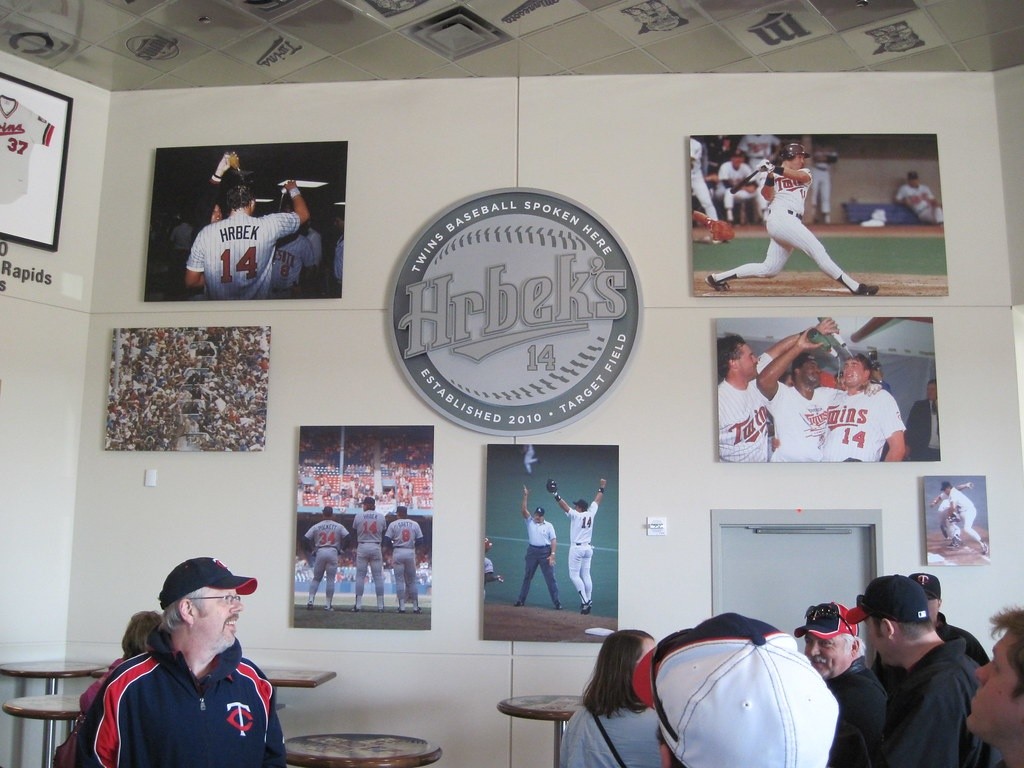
[704,217,734,241]
[547,478,557,492]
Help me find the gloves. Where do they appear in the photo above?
[497,575,504,583]
[757,159,776,174]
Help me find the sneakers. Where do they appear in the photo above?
[855,282,879,297]
[946,536,988,554]
[308,600,423,614]
[580,600,593,615]
[513,598,561,610]
[705,274,732,291]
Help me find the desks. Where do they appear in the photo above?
[1,661,110,768]
[496,695,584,768]
[260,668,337,711]
[284,734,442,768]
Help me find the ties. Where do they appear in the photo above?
[931,401,939,435]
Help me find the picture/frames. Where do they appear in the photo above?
[0,72,73,253]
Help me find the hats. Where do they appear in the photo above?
[909,573,940,602]
[730,149,744,157]
[632,611,839,768]
[846,575,931,624]
[793,602,859,641]
[320,495,407,517]
[161,557,258,608]
[940,481,950,491]
[485,537,493,550]
[534,499,590,515]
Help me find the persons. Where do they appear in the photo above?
[150,150,344,299]
[688,135,943,298]
[795,603,890,768]
[58,557,288,768]
[106,327,272,451]
[930,481,989,555]
[482,478,607,615]
[632,612,839,768]
[298,427,433,614]
[717,317,942,461]
[845,572,1024,768]
[559,630,659,768]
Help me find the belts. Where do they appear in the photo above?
[768,207,803,220]
[530,542,590,549]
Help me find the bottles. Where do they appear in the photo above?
[808,328,839,358]
[817,317,847,350]
[222,149,244,178]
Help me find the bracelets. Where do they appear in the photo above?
[599,488,604,493]
[556,496,561,502]
[551,552,555,555]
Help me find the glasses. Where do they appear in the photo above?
[185,592,241,607]
[806,606,855,639]
[856,594,896,623]
[650,628,693,740]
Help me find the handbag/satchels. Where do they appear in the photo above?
[52,711,91,768]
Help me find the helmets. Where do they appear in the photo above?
[778,143,811,162]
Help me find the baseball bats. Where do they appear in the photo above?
[730,162,769,194]
